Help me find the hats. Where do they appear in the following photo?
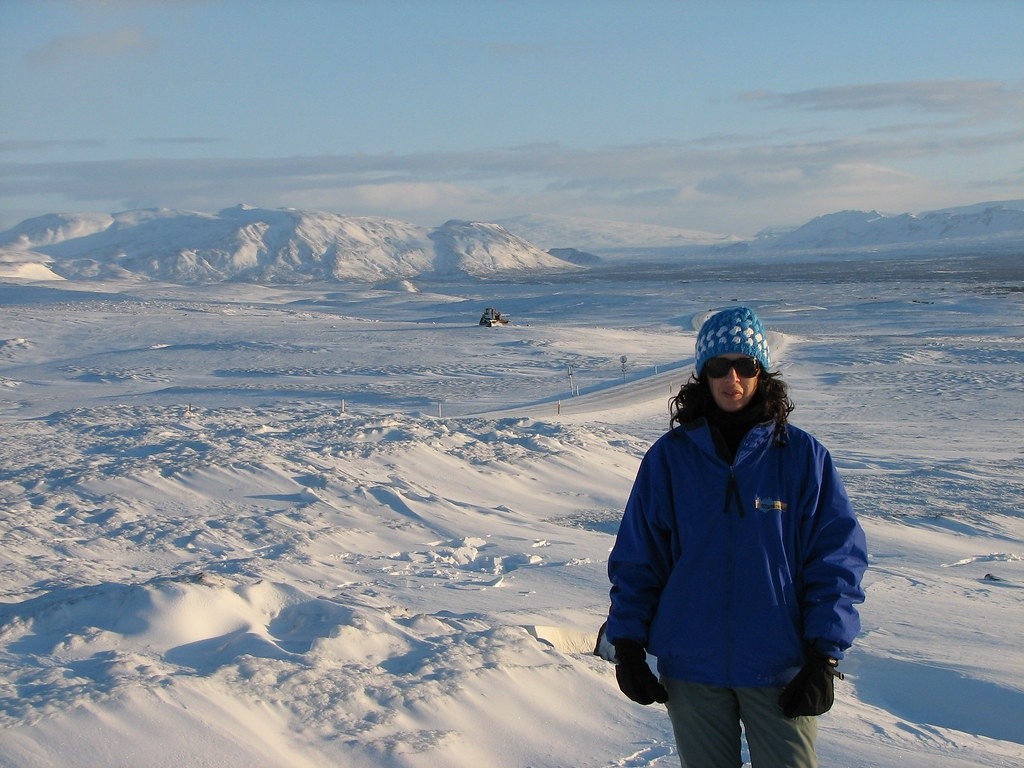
[695,307,773,378]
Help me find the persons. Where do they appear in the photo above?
[594,305,869,768]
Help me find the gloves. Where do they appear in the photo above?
[777,654,845,718]
[612,637,669,705]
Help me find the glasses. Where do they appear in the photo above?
[705,356,762,378]
